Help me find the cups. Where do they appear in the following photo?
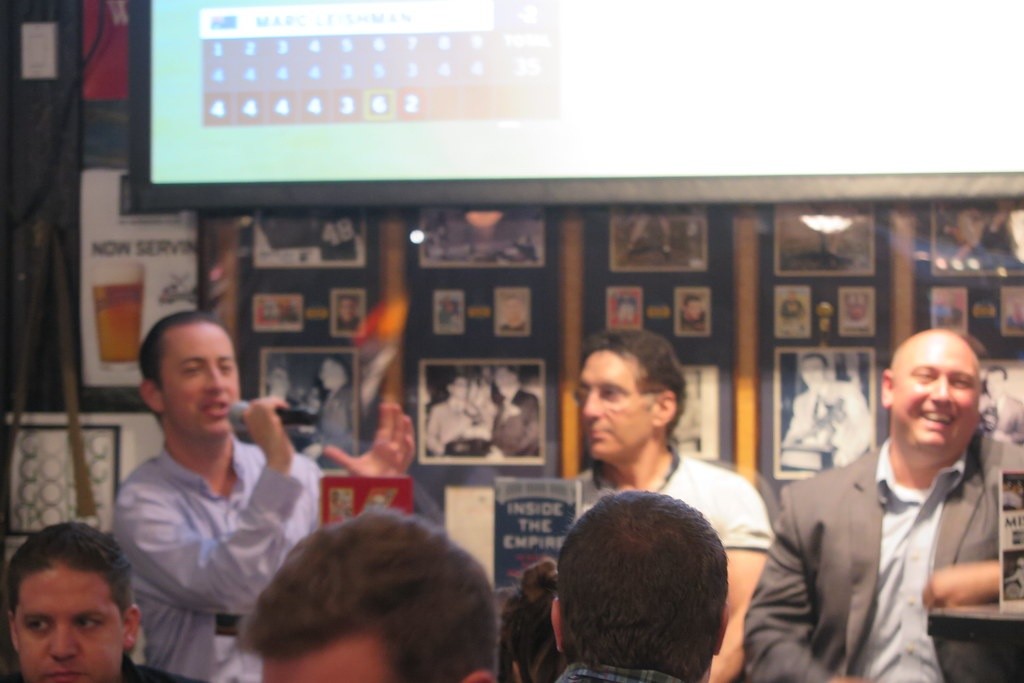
[93,261,145,362]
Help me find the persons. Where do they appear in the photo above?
[498,330,773,683]
[0,521,209,683]
[338,296,360,330]
[267,355,350,472]
[934,290,961,326]
[1008,300,1024,330]
[680,293,705,332]
[781,293,805,335]
[618,217,675,262]
[845,292,870,330]
[238,506,497,683]
[500,294,525,334]
[428,367,540,457]
[782,353,870,466]
[976,363,1024,445]
[744,328,1024,683]
[114,311,413,683]
[615,291,637,330]
[937,197,1024,270]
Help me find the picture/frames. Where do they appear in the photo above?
[608,208,710,271]
[771,201,877,277]
[608,285,1024,340]
[330,288,366,339]
[253,293,302,332]
[433,289,465,336]
[251,209,367,266]
[930,200,1024,279]
[979,359,1024,447]
[773,346,875,482]
[416,207,546,270]
[493,287,528,337]
[418,357,548,466]
[258,347,359,476]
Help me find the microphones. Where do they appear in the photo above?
[229,399,321,435]
